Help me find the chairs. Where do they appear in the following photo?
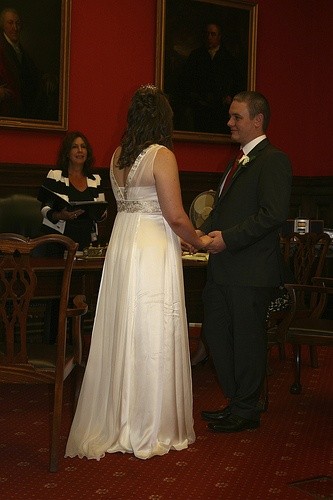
[250,228,333,412]
[0,234,89,487]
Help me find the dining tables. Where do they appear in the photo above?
[0,241,333,345]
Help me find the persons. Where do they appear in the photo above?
[40,131,107,345]
[64,84,213,460]
[181,91,293,432]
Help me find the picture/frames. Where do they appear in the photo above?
[151,0,260,145]
[0,0,74,133]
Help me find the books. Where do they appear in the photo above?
[37,185,107,221]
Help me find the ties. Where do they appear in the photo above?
[220,149,245,195]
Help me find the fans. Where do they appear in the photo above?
[188,187,224,231]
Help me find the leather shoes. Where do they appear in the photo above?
[201,405,261,432]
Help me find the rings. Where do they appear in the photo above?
[75,214,76,216]
